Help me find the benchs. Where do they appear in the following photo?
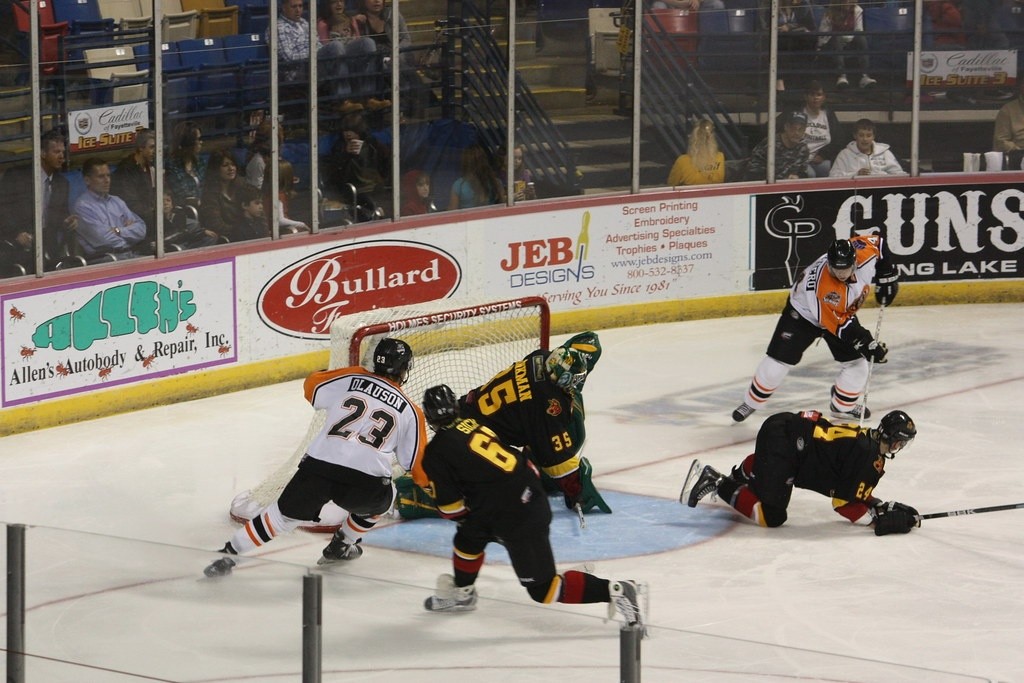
[0,0,1024,280]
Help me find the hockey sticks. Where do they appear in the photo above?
[858,296,887,429]
[919,502,1024,520]
[575,502,586,530]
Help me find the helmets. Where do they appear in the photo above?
[373,338,413,386]
[877,410,916,454]
[423,385,459,427]
[544,346,588,388]
[827,237,856,277]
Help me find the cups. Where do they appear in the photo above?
[352,139,364,154]
[858,157,871,174]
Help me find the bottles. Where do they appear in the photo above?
[525,182,537,200]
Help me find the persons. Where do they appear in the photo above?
[679,410,920,536]
[425,384,649,641]
[204,338,429,576]
[393,331,613,520]
[648,0,1024,177]
[0,0,537,278]
[731,233,898,426]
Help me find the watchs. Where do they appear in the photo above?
[114,227,120,235]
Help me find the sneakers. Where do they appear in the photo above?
[204,543,239,581]
[727,463,752,486]
[423,572,478,612]
[602,578,650,640]
[859,74,877,88]
[338,101,364,114]
[829,386,871,422]
[834,73,850,88]
[317,526,363,565]
[732,403,755,426]
[366,98,392,111]
[679,459,732,508]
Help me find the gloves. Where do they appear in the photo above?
[851,330,888,364]
[874,269,898,306]
[555,470,582,509]
[871,498,919,536]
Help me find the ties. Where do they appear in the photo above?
[42,179,50,229]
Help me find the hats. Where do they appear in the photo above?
[340,113,362,135]
[786,111,808,125]
